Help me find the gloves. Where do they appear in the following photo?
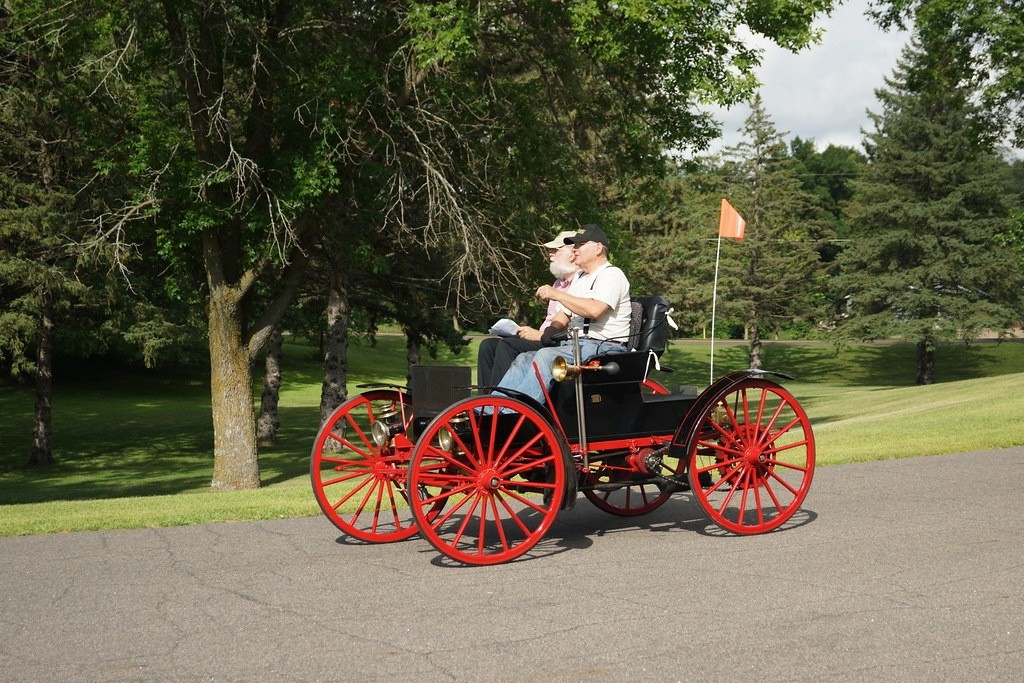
[541,326,558,348]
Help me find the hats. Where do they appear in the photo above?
[543,231,577,248]
[563,224,608,246]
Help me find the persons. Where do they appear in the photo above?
[473,225,632,416]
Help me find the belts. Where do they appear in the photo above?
[569,337,628,348]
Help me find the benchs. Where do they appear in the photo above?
[579,294,670,383]
